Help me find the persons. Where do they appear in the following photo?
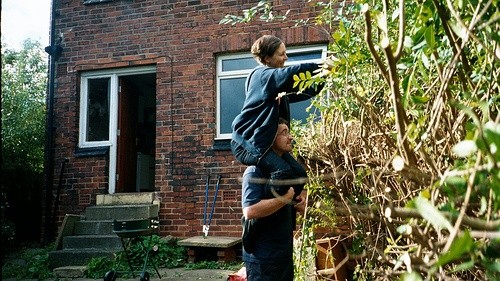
[230,36,327,199]
[239,118,307,281]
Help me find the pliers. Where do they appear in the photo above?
[202,175,220,238]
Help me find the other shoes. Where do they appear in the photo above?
[240,215,258,255]
